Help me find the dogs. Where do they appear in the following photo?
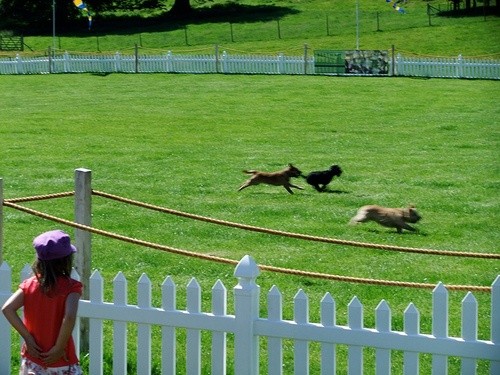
[347,203,422,234]
[238,162,305,195]
[300,165,344,193]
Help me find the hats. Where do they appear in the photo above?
[33,230,77,262]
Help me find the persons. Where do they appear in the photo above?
[2,229,83,375]
